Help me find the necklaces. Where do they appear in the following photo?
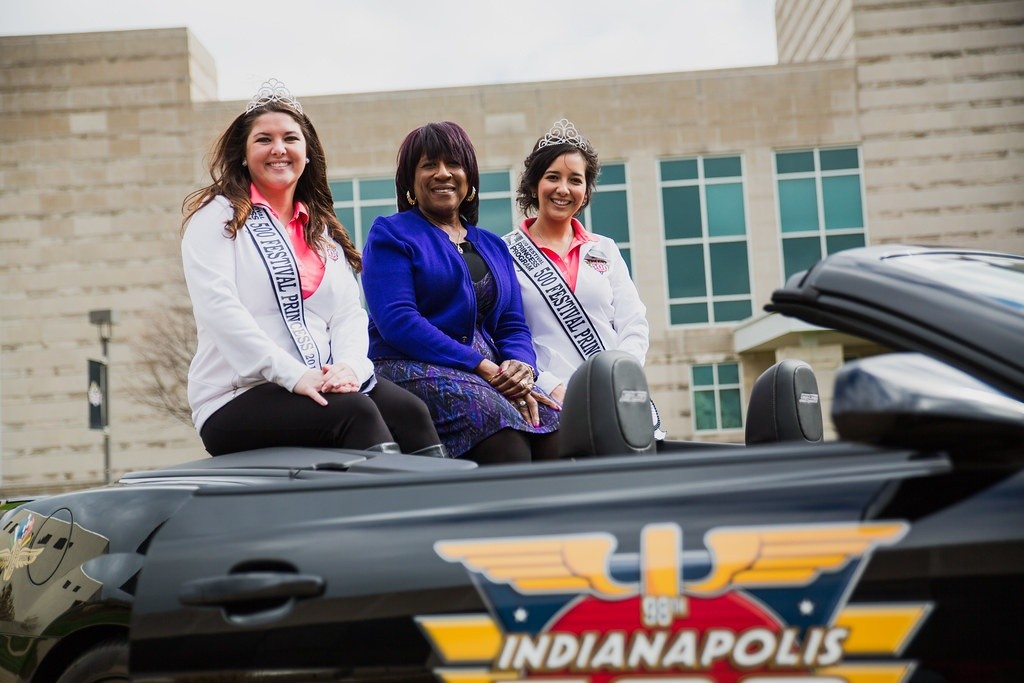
[432,220,465,254]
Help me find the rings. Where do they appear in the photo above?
[518,401,526,409]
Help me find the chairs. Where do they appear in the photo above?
[557,350,657,462]
[745,358,824,450]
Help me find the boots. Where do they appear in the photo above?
[365,442,401,454]
[412,444,445,458]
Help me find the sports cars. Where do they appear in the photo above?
[2,236,1024,683]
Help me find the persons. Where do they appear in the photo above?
[360,121,571,469]
[500,119,666,440]
[178,78,449,461]
